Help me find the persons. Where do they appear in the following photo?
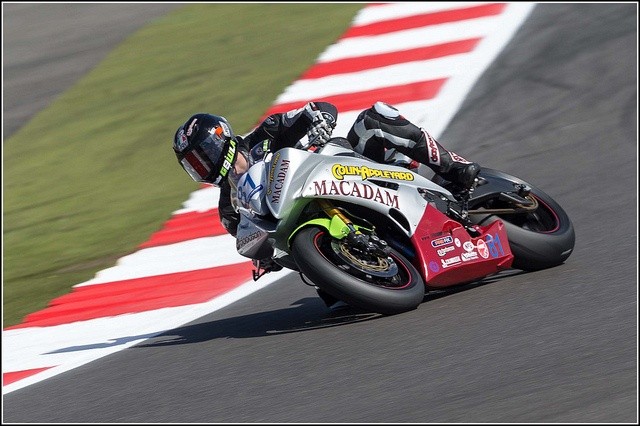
[173,99,481,309]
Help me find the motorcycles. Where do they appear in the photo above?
[228,101,576,309]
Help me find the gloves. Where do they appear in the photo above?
[308,120,333,147]
[253,258,278,271]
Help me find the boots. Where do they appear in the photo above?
[410,128,481,188]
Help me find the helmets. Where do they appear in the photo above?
[173,114,239,186]
